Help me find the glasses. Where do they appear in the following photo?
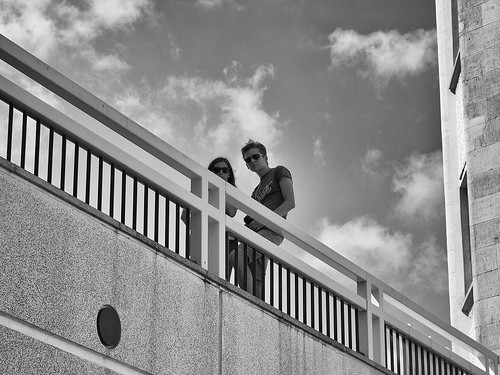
[243,153,264,162]
[212,166,228,174]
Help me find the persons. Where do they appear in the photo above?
[181,157,237,281]
[229,138,295,302]
